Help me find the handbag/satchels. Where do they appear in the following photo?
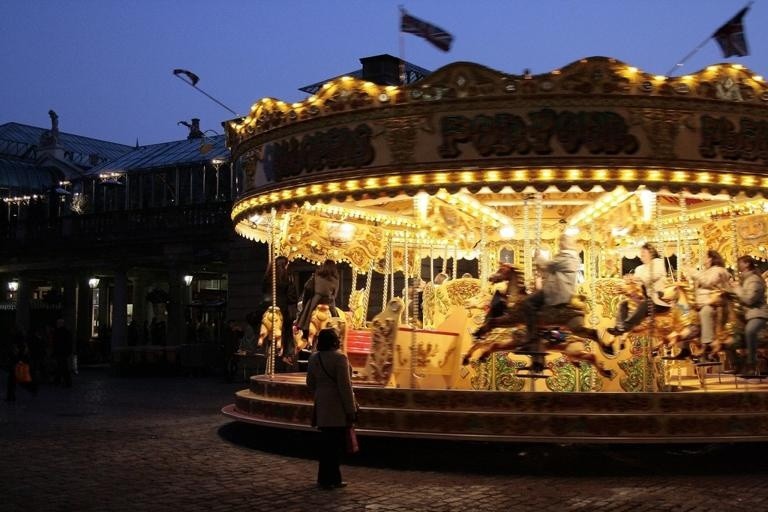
[345,396,362,423]
[344,425,360,457]
[304,272,316,299]
[15,362,32,383]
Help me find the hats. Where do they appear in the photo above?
[314,327,342,340]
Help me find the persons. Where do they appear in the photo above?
[223,319,243,377]
[3,323,51,402]
[461,272,472,278]
[433,271,449,284]
[296,258,341,341]
[604,239,768,377]
[128,314,166,346]
[261,255,299,368]
[304,329,359,490]
[514,226,583,353]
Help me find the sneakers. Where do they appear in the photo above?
[232,349,250,356]
[319,480,348,488]
[723,365,760,378]
[607,327,625,337]
[696,344,714,359]
[672,349,692,358]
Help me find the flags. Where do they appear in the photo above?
[712,8,751,59]
[399,12,455,51]
[176,69,200,86]
